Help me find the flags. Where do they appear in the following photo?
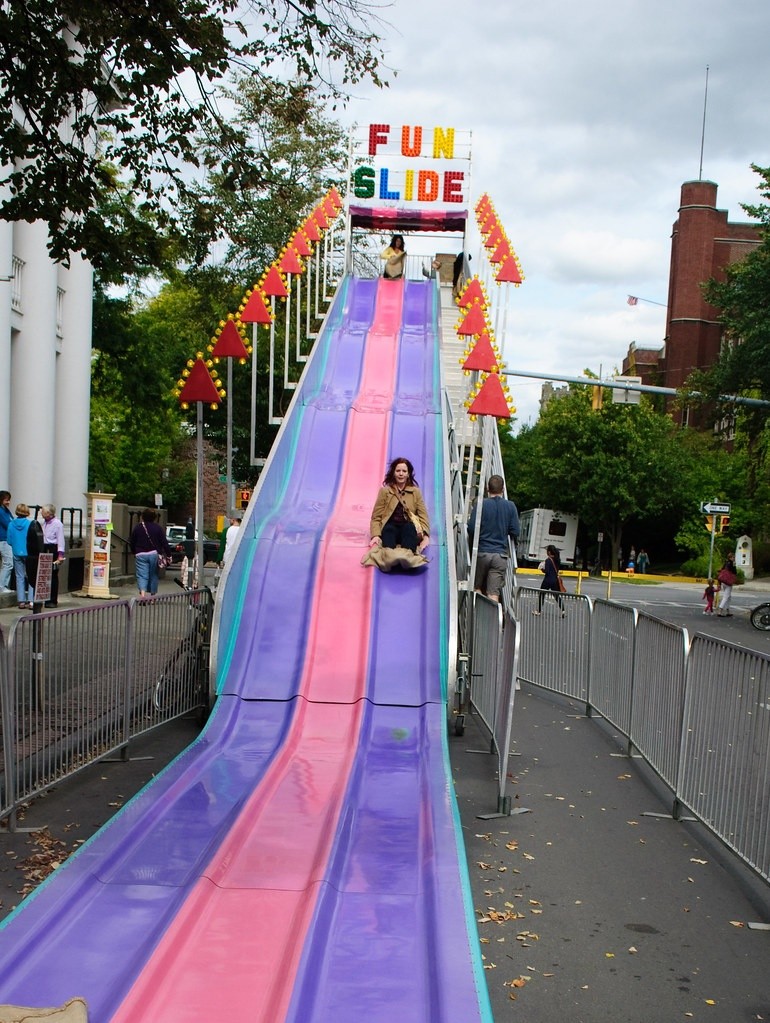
[627,295,638,306]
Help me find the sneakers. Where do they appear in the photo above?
[29,602,33,609]
[711,612,714,615]
[532,611,540,616]
[562,611,565,619]
[703,610,708,616]
[19,602,25,608]
[0,587,14,594]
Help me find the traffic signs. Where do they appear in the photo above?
[701,502,731,514]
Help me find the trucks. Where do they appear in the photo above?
[516,508,579,568]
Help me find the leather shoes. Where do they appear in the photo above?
[717,613,733,617]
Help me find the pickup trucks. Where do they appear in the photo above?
[179,540,220,565]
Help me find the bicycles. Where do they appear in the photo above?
[152,577,215,714]
[750,603,770,631]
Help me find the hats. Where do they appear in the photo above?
[225,510,241,519]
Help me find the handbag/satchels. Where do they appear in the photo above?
[410,512,425,543]
[212,566,220,592]
[715,592,721,609]
[717,560,737,586]
[158,554,172,569]
[557,575,566,592]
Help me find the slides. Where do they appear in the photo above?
[0,271,495,1023]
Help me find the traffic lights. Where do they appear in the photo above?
[720,516,731,533]
[704,516,713,532]
[236,492,249,508]
[217,516,231,532]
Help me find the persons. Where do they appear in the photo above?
[368,458,432,572]
[379,235,407,278]
[452,252,471,287]
[532,545,567,619]
[216,510,243,569]
[618,546,650,574]
[129,508,173,606]
[0,491,65,609]
[467,475,521,603]
[421,260,442,279]
[702,553,738,617]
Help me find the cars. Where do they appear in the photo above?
[166,526,206,547]
[167,539,185,563]
